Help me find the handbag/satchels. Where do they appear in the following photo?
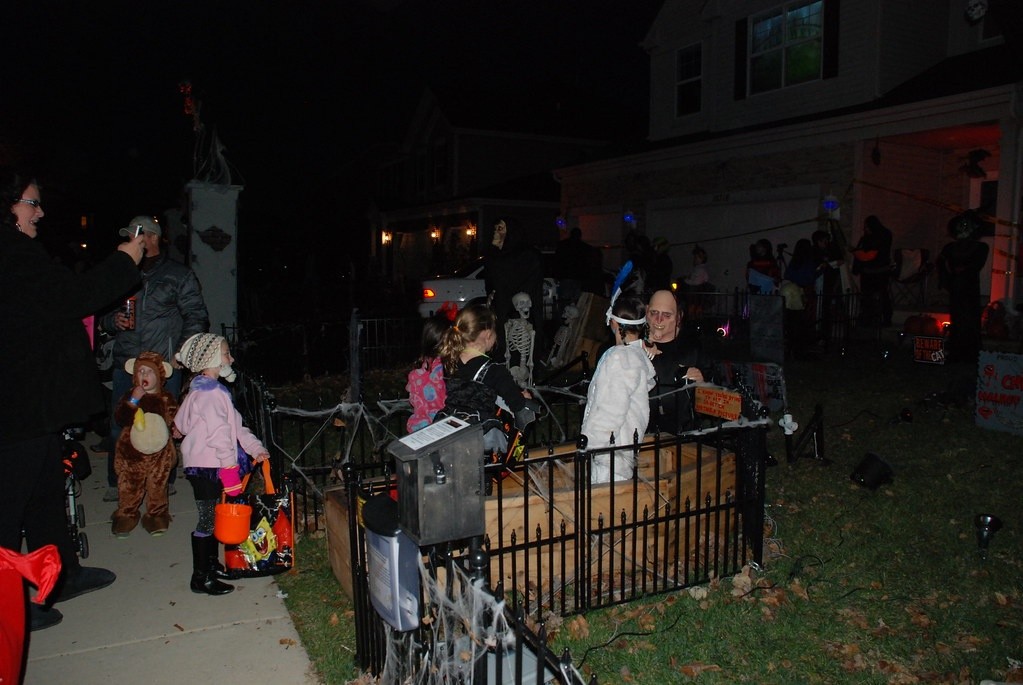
[222,454,295,577]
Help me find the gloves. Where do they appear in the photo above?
[219,464,243,496]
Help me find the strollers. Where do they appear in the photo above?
[62,416,88,558]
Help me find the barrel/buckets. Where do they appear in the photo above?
[214,487,252,545]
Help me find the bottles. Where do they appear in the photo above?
[122,296,136,330]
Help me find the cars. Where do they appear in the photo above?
[417,252,571,324]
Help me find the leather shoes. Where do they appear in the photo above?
[90,438,110,453]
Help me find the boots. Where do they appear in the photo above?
[25,601,63,632]
[191,531,233,594]
[212,531,241,580]
[29,550,115,604]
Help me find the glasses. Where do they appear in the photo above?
[16,198,43,208]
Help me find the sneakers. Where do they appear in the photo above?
[104,486,121,501]
[167,484,177,496]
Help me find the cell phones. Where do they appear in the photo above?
[134,225,142,239]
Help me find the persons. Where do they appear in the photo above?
[432,305,533,493]
[537,207,992,337]
[576,298,659,488]
[174,331,272,596]
[110,350,182,539]
[597,290,714,436]
[402,298,458,435]
[0,171,149,631]
[98,214,210,502]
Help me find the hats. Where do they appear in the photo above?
[174,333,237,383]
[118,215,161,235]
